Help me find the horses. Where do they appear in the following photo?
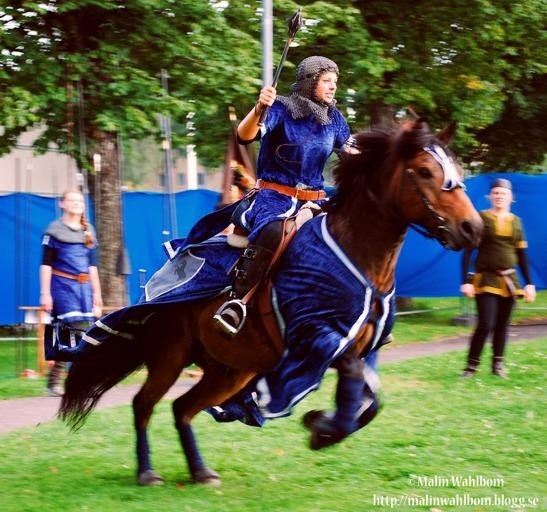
[54,103,489,489]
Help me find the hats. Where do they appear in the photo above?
[491,179,511,190]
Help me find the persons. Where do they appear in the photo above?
[221,56,360,327]
[40,191,103,396]
[460,178,536,380]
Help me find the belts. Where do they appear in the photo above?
[53,270,92,284]
[256,179,326,200]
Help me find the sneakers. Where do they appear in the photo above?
[48,384,65,396]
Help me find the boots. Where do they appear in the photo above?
[221,244,273,327]
